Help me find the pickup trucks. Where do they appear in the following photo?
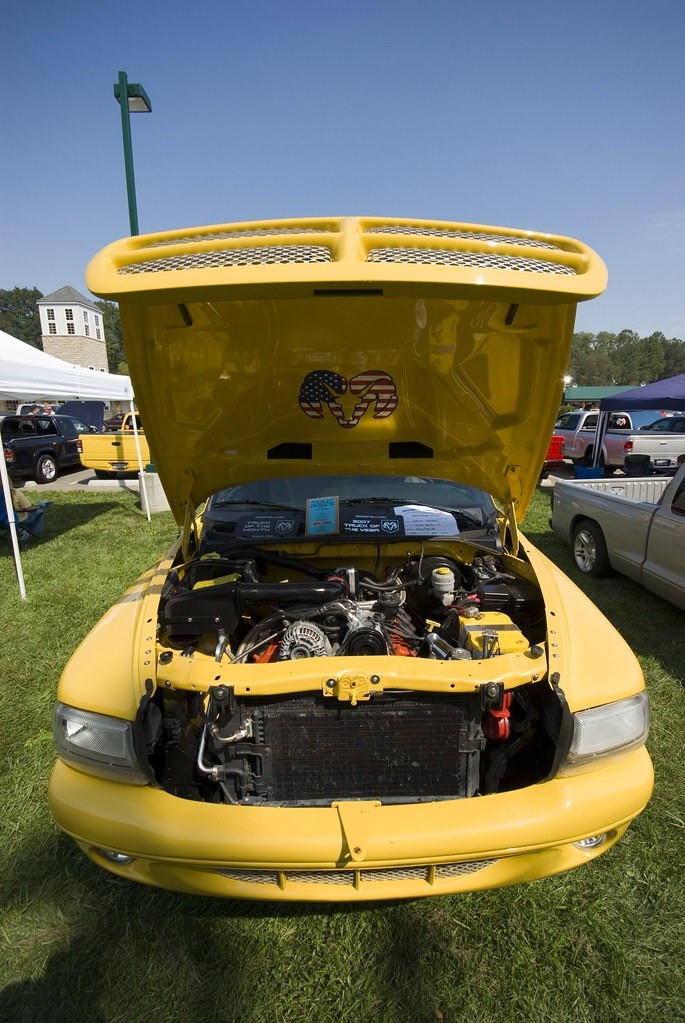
[548,461,685,613]
[0,401,105,483]
[76,412,151,479]
[552,411,685,474]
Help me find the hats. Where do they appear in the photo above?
[29,405,41,411]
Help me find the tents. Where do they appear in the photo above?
[0,329,152,598]
[592,374,685,471]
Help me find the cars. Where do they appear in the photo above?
[48,215,655,906]
[640,417,685,434]
[540,436,565,479]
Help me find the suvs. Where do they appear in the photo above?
[103,413,142,431]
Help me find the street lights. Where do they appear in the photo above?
[112,70,153,237]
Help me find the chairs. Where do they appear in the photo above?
[21,424,33,433]
[4,420,19,433]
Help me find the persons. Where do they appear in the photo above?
[43,404,55,415]
[27,405,41,415]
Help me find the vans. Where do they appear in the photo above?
[16,404,62,417]
